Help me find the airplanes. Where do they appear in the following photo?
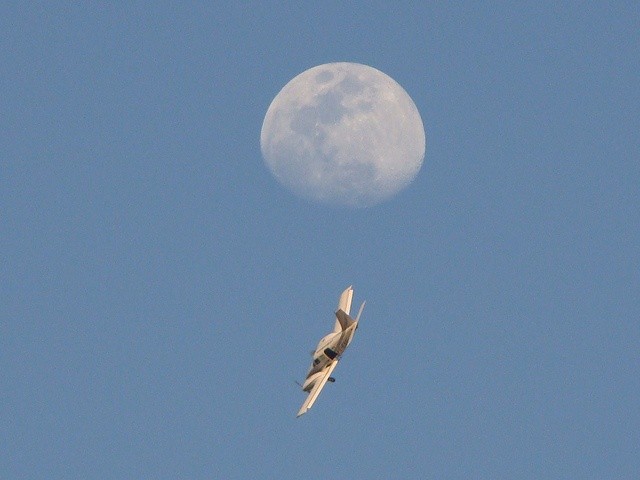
[295,284,367,418]
